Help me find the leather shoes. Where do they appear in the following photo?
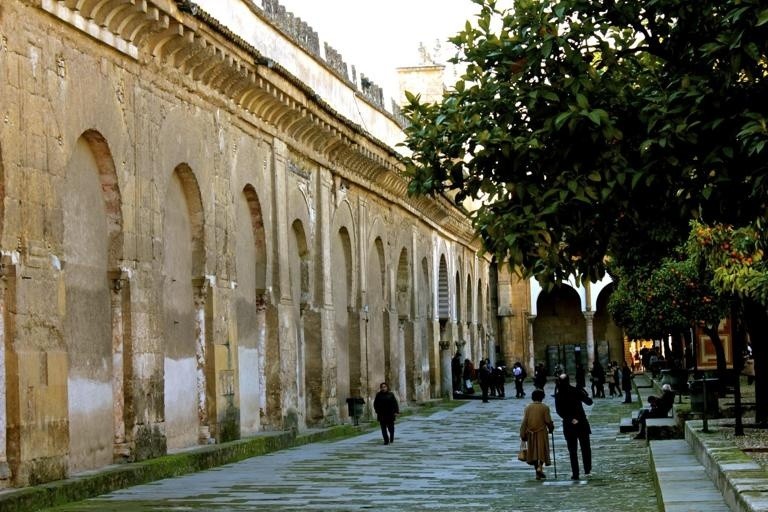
[536,467,545,478]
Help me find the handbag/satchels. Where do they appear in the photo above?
[517,444,527,461]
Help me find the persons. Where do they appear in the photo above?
[373,382,400,445]
[554,372,594,481]
[462,357,623,403]
[620,361,633,403]
[519,390,555,480]
[632,382,675,440]
[451,352,464,395]
[640,344,649,366]
